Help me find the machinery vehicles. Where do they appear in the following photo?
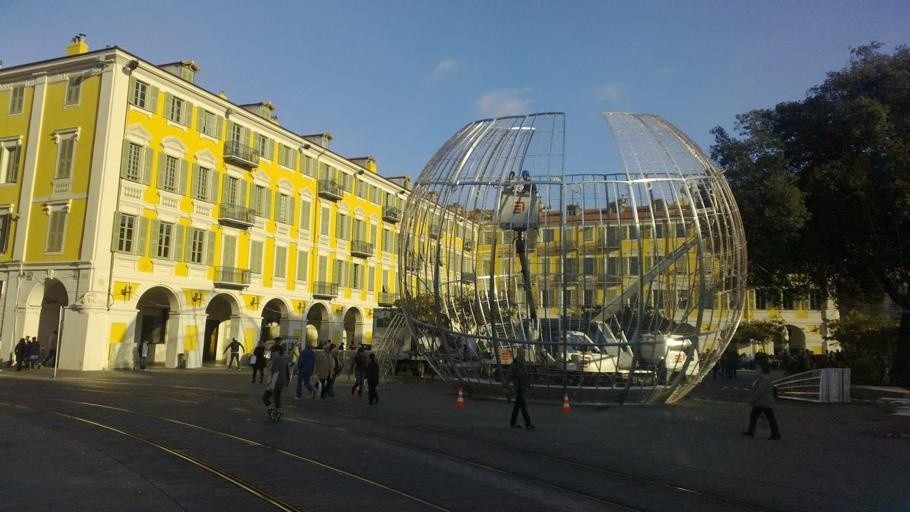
[555,217,725,384]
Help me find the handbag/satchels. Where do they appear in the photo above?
[772,386,780,401]
[249,355,257,365]
[311,367,319,384]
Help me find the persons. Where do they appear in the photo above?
[504,345,537,432]
[14,330,58,373]
[262,344,290,420]
[709,345,843,381]
[249,333,381,405]
[223,337,245,372]
[138,339,149,369]
[502,169,537,196]
[738,359,781,440]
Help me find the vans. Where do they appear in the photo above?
[250,339,298,367]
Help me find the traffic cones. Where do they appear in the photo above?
[456,384,465,409]
[560,385,573,413]
[353,384,360,396]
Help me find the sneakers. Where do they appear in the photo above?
[311,389,316,400]
[766,434,781,441]
[527,424,537,432]
[293,396,301,400]
[741,430,754,440]
[509,423,522,429]
